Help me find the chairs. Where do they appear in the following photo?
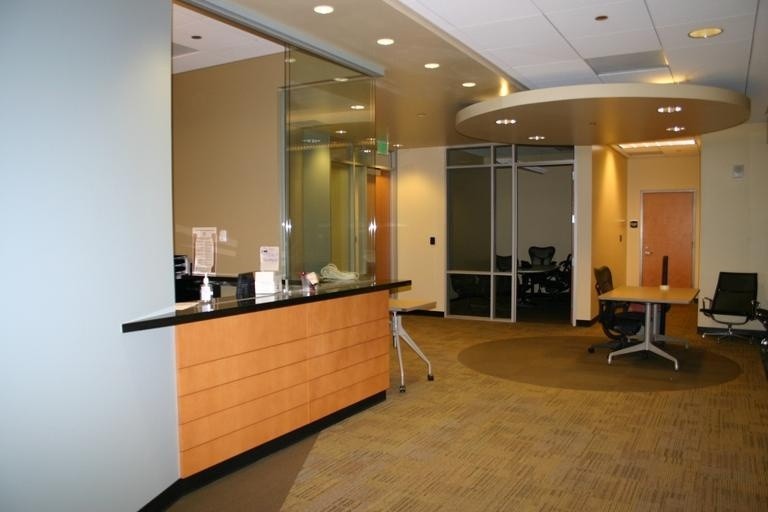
[700,272,760,346]
[497,246,572,307]
[588,265,646,353]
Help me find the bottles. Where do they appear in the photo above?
[199,272,214,304]
[661,256,669,290]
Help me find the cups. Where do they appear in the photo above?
[300,271,316,290]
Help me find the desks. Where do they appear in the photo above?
[597,284,701,371]
[388,298,435,393]
